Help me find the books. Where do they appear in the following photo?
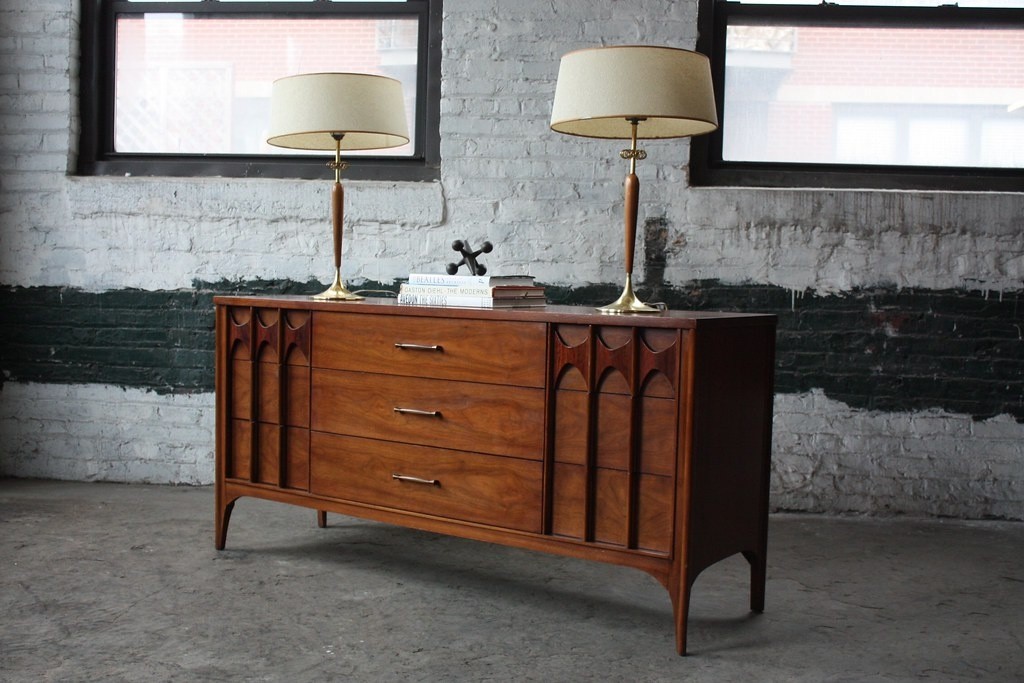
[397,271,549,310]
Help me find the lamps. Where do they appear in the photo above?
[267,72,411,301]
[550,45,718,314]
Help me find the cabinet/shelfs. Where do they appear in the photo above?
[215,295,779,656]
